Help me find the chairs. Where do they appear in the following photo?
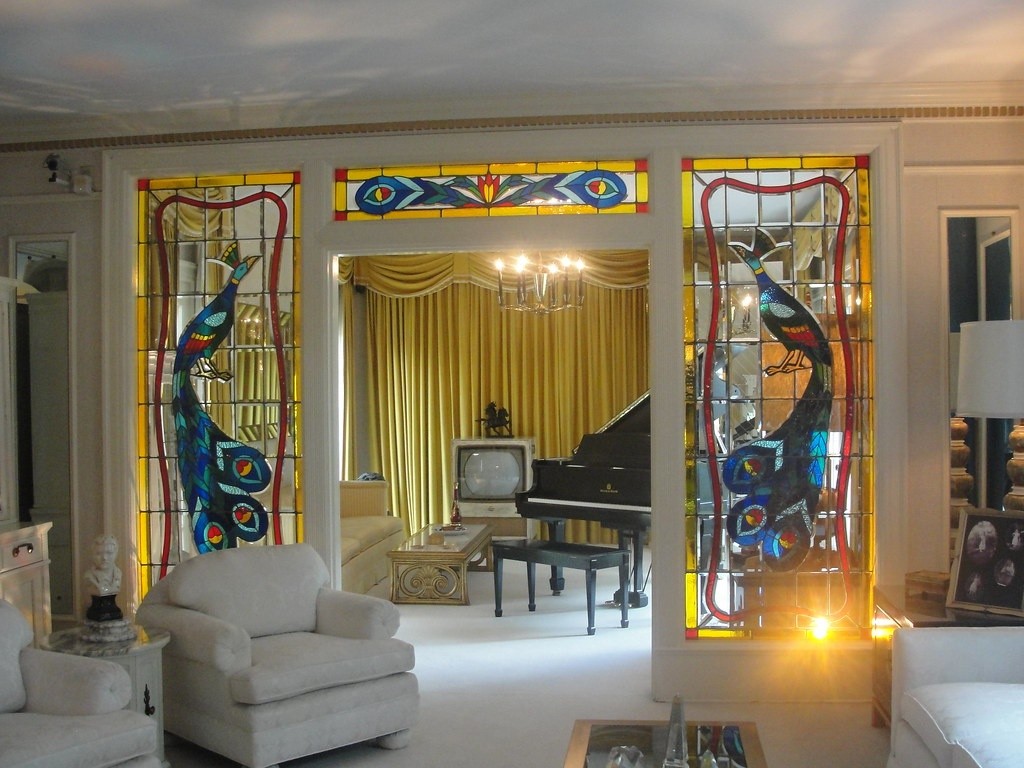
[134,542,423,768]
[0,598,163,768]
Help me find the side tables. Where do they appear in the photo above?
[40,622,171,767]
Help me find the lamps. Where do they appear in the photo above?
[954,320,1023,510]
[493,252,589,314]
[43,151,94,197]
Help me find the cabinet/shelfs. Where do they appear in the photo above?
[1,520,52,642]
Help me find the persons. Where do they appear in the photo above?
[84,533,125,596]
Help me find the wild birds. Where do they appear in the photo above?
[171,240,275,554]
[727,226,836,575]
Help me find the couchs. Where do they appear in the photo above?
[886,626,1024,768]
[340,480,407,593]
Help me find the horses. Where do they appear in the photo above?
[475,407,512,438]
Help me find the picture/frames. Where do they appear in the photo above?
[944,507,1024,619]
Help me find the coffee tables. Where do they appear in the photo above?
[386,522,496,607]
[563,719,768,768]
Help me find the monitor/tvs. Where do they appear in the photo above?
[451,441,530,504]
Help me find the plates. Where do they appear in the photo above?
[434,527,467,536]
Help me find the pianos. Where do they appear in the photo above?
[515,342,747,615]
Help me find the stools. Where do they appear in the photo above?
[489,538,633,636]
